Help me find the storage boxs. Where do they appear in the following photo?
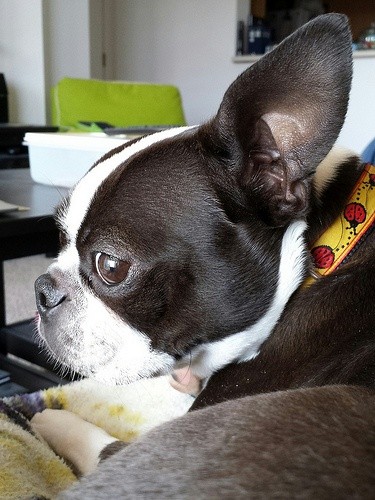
[22,132,130,188]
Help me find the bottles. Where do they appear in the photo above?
[248,17,269,55]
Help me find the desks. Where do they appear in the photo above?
[0,168,77,380]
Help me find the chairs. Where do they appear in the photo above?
[50,77,186,127]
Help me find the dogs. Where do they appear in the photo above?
[33,12,374,500]
